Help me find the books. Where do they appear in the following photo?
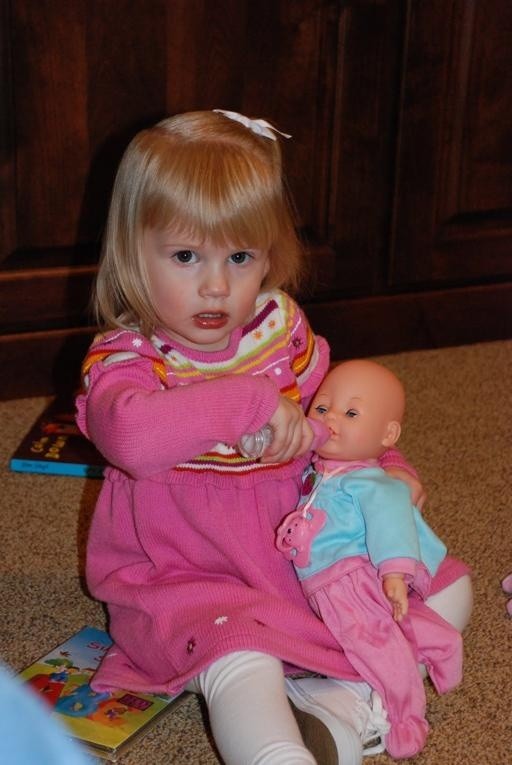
[13,623,198,763]
[10,397,106,479]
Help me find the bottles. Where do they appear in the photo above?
[235,416,330,464]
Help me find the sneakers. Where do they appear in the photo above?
[285,676,391,765]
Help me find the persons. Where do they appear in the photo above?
[275,357,467,759]
[81,107,473,765]
